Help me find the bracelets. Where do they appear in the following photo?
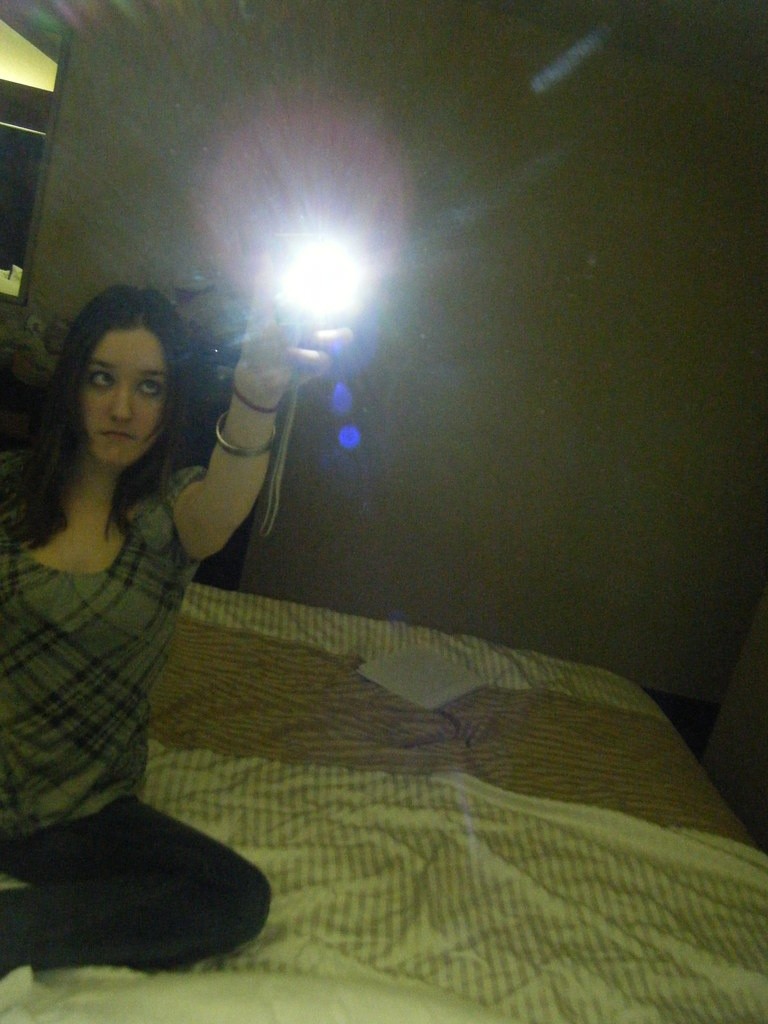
[215,410,277,457]
[227,374,281,413]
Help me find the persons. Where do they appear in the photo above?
[0,241,370,983]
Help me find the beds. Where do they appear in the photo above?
[0,582,768,1024]
